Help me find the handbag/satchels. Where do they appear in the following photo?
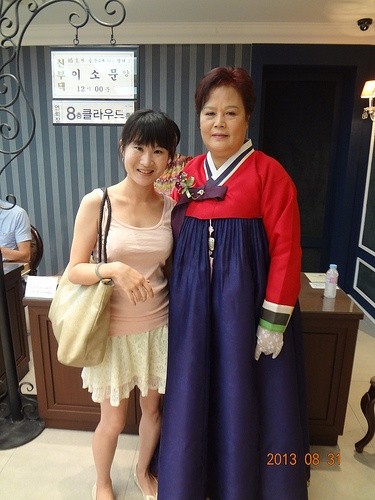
[47,186,116,367]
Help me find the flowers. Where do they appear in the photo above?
[174,172,198,198]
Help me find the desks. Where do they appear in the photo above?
[22,270,364,447]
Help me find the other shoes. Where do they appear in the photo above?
[134,465,160,500]
[93,478,112,500]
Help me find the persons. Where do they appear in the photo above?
[0,199,32,274]
[147,64,311,500]
[67,107,182,500]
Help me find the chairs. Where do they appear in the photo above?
[30,224,44,276]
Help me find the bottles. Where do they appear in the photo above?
[323,264,339,298]
[322,297,335,311]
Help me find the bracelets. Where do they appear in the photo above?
[96,262,106,280]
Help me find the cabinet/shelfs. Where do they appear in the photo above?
[0,263,30,397]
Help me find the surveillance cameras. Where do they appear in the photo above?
[358,18,372,31]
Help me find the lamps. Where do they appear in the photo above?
[360,80,375,122]
